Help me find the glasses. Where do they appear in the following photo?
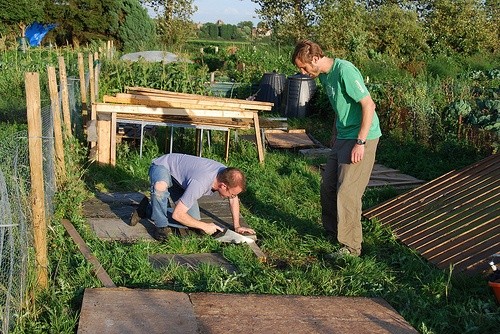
[226,188,237,198]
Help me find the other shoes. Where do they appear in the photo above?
[325,246,361,257]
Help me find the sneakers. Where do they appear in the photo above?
[129,196,149,226]
[154,227,168,241]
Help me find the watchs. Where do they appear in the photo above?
[355,138,366,145]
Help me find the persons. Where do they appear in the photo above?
[129,153,258,236]
[291,41,383,261]
